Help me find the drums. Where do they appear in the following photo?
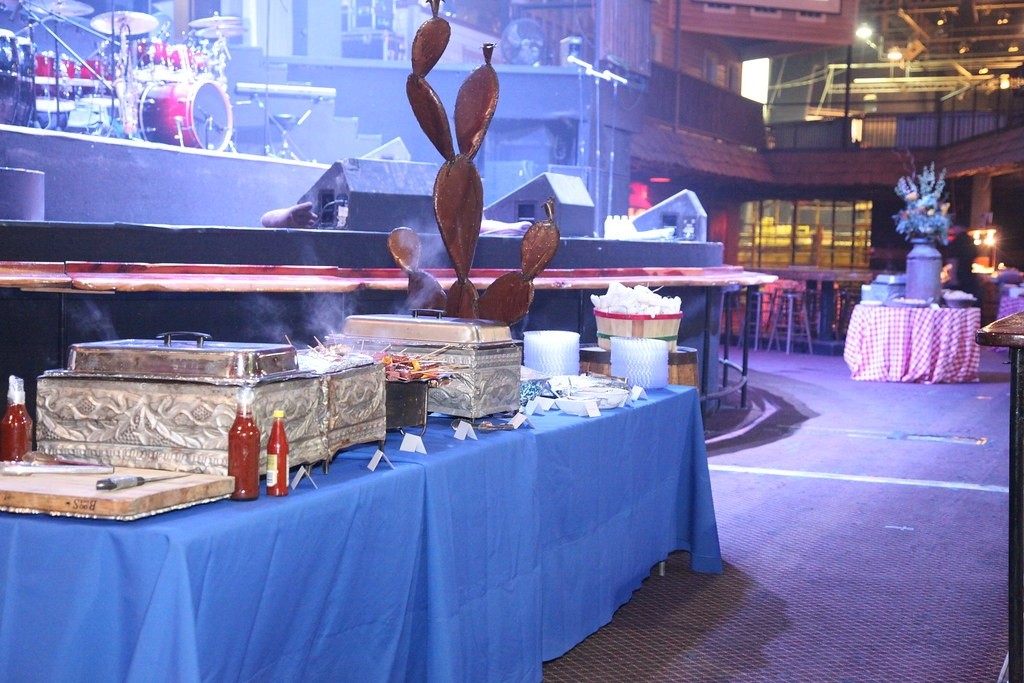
[194,52,208,81]
[12,36,37,126]
[33,51,76,113]
[170,48,198,74]
[80,58,122,107]
[137,77,233,151]
[0,29,21,125]
[128,39,171,81]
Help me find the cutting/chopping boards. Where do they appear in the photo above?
[0,464,236,517]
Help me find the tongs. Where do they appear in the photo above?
[0,459,114,474]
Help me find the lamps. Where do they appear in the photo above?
[967,213,1000,246]
[856,10,1019,90]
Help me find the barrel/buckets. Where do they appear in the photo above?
[593,306,683,353]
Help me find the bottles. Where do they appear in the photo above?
[228,388,261,501]
[0,375,33,462]
[266,411,290,496]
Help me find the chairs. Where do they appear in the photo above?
[267,95,323,163]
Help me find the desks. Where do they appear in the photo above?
[976,311,1024,683]
[0,261,73,452]
[339,260,780,436]
[997,295,1024,319]
[843,305,984,384]
[0,384,723,683]
[65,260,361,435]
[746,268,880,354]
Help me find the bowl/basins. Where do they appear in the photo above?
[576,387,629,409]
[555,395,602,416]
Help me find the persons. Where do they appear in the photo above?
[990,260,1023,285]
[945,220,974,292]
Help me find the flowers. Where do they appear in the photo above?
[891,160,953,246]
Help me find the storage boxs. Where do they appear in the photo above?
[872,274,906,299]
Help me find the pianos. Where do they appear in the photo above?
[235,82,337,161]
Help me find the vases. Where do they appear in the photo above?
[907,239,943,308]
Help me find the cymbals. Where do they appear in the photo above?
[24,0,94,16]
[195,26,247,38]
[189,16,242,26]
[90,11,159,35]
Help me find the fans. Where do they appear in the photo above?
[500,18,546,66]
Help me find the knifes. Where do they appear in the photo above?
[95,471,193,492]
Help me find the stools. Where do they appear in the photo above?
[725,286,863,354]
